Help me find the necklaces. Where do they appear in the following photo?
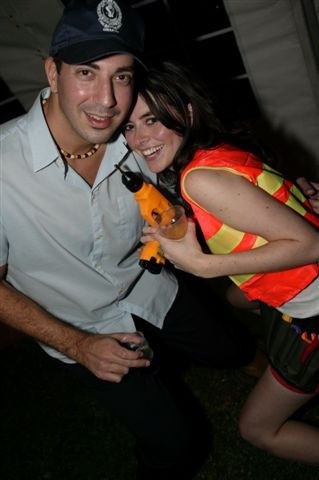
[58,142,101,160]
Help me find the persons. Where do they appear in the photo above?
[0,0,319,479]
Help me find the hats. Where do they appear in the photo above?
[50,0,150,72]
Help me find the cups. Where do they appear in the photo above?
[156,205,188,239]
[120,331,154,361]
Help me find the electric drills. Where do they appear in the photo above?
[114,164,183,276]
